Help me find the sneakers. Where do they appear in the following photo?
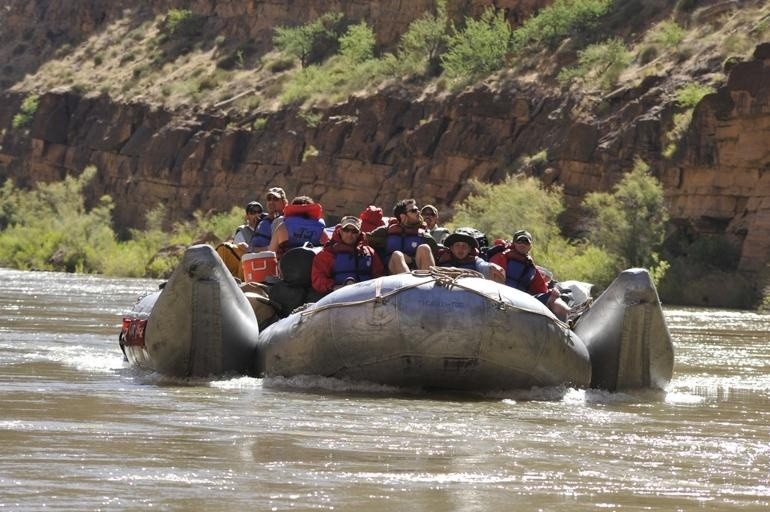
[566,297,593,323]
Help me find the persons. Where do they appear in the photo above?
[232,186,573,314]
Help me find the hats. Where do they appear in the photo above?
[340,216,362,230]
[420,205,439,214]
[444,228,479,248]
[246,201,263,212]
[513,230,532,243]
[265,187,287,199]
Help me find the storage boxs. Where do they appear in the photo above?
[242,250,278,282]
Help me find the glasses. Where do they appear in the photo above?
[342,228,358,234]
[268,197,278,201]
[518,240,528,244]
[408,209,420,213]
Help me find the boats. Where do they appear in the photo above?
[120,243,677,397]
